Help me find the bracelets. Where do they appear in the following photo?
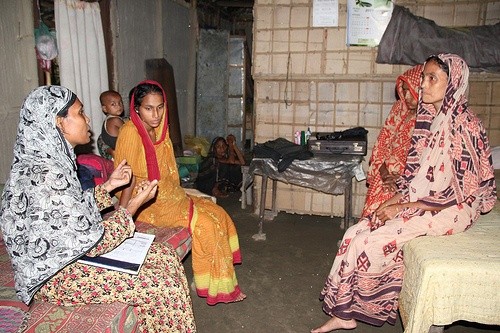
[394,205,399,214]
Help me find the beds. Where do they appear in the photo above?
[0,157,213,333]
[398,147,500,333]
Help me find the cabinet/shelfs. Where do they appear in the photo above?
[198,37,245,159]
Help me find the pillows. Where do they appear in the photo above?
[0,286,28,332]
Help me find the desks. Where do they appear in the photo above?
[245,155,361,232]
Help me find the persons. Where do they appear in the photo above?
[310,52,498,333]
[0,86,197,333]
[113,79,247,306]
[337,64,423,250]
[97,90,246,210]
